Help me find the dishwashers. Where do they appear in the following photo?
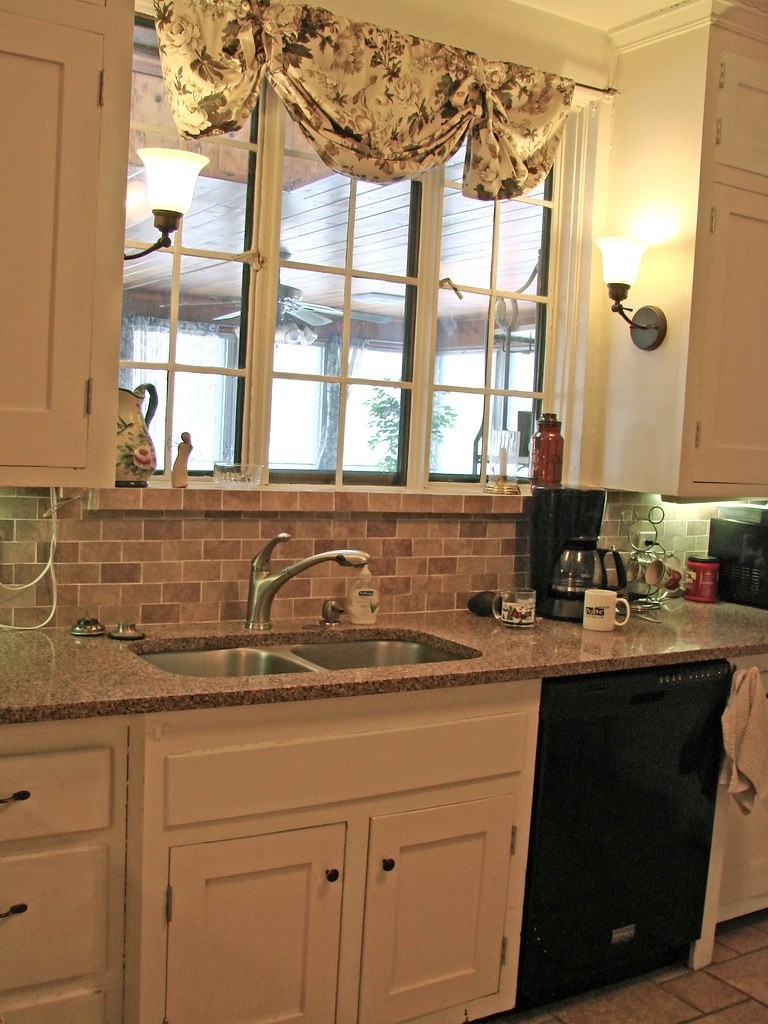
[514,657,736,1010]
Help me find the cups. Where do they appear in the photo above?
[583,589,632,632]
[492,588,537,629]
[214,461,264,488]
[483,430,522,496]
[626,549,683,592]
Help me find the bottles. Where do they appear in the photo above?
[683,554,721,603]
[529,413,564,492]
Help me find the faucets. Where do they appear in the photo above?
[320,599,345,626]
[245,532,372,631]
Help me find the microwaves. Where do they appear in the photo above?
[706,517,768,609]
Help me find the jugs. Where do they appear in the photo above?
[114,383,158,488]
[546,531,626,600]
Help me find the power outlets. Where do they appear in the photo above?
[637,532,658,549]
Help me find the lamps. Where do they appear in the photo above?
[596,234,670,351]
[125,146,211,264]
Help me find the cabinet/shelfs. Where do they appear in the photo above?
[141,681,546,1024]
[0,1,133,490]
[612,0,768,497]
[0,709,138,1024]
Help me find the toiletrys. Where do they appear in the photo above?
[346,563,381,624]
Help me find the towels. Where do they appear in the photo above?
[717,665,768,816]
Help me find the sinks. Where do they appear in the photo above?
[276,639,470,670]
[138,646,323,676]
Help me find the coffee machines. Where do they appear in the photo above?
[530,483,608,623]
